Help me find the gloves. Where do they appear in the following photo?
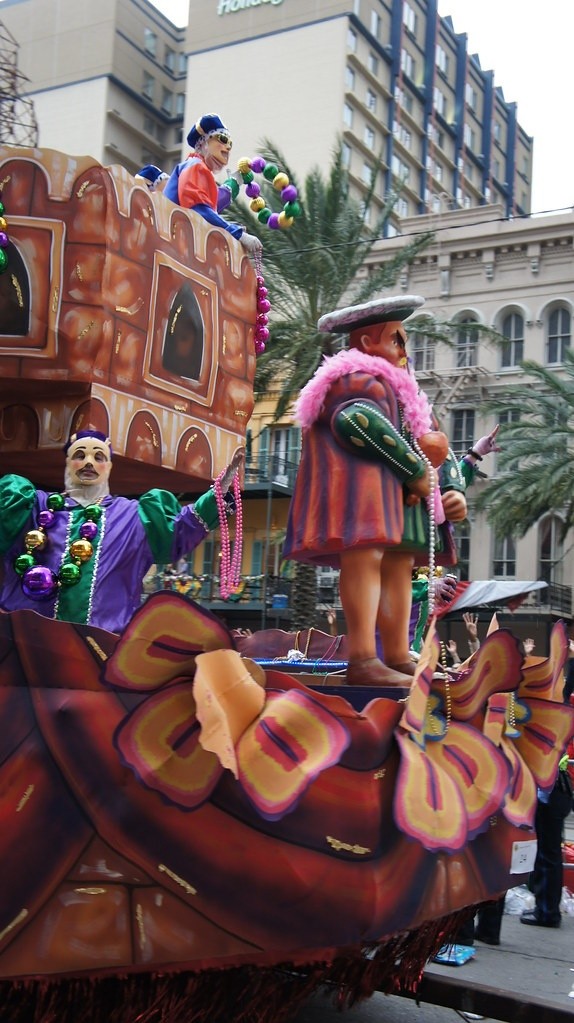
[239,232,263,253]
[231,167,244,185]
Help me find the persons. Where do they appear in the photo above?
[135,165,170,191]
[0,431,245,635]
[462,612,479,654]
[446,639,462,664]
[174,558,189,576]
[520,771,574,927]
[163,113,263,252]
[282,294,469,687]
[460,895,506,946]
[523,638,535,656]
[164,563,176,575]
[411,423,501,606]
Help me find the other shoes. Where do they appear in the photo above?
[474,926,500,945]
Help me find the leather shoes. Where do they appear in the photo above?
[520,913,562,927]
[522,909,536,915]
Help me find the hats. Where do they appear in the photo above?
[63,431,112,462]
[186,114,231,150]
[135,165,163,185]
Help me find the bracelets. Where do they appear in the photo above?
[451,654,458,658]
[467,447,483,462]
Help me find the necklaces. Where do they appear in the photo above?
[15,493,102,601]
[294,347,445,614]
[215,468,243,599]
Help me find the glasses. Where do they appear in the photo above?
[212,134,233,150]
